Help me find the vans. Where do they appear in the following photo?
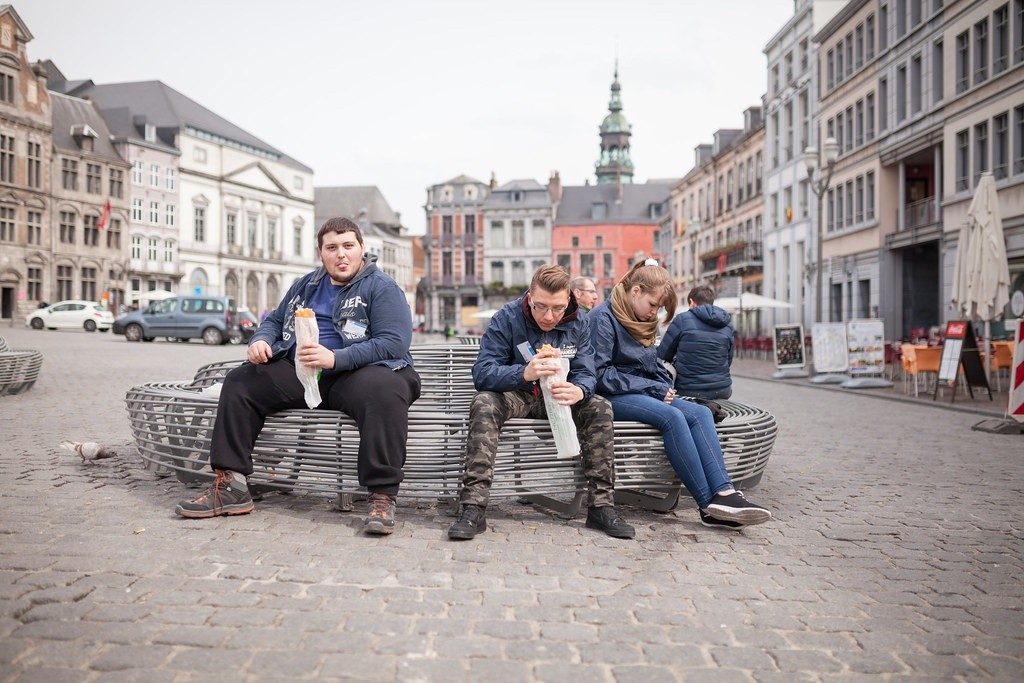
[112,296,238,345]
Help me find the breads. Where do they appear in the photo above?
[295,307,315,318]
[536,344,560,357]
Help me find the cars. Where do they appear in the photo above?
[166,307,258,344]
[25,300,115,332]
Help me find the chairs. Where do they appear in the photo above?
[885,328,1014,382]
[734,336,811,362]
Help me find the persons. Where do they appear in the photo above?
[778,333,802,364]
[447,264,637,540]
[565,278,598,317]
[584,255,772,533]
[174,218,420,535]
[656,285,733,401]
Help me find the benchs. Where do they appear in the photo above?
[0,336,45,396]
[124,336,778,522]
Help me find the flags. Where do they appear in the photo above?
[94,199,111,228]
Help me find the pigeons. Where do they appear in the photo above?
[60,440,118,466]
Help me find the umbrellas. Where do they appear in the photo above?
[952,171,1011,385]
[714,291,796,337]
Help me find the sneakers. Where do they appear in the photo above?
[707,490,771,525]
[364,493,397,534]
[174,469,255,518]
[585,505,635,537]
[449,506,487,538]
[698,508,750,529]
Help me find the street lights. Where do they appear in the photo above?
[803,137,841,322]
[608,268,615,292]
[686,218,700,288]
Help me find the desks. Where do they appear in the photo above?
[901,342,1013,398]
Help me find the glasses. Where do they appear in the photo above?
[580,290,596,296]
[530,297,569,314]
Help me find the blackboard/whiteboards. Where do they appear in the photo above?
[774,323,806,368]
[938,320,990,386]
